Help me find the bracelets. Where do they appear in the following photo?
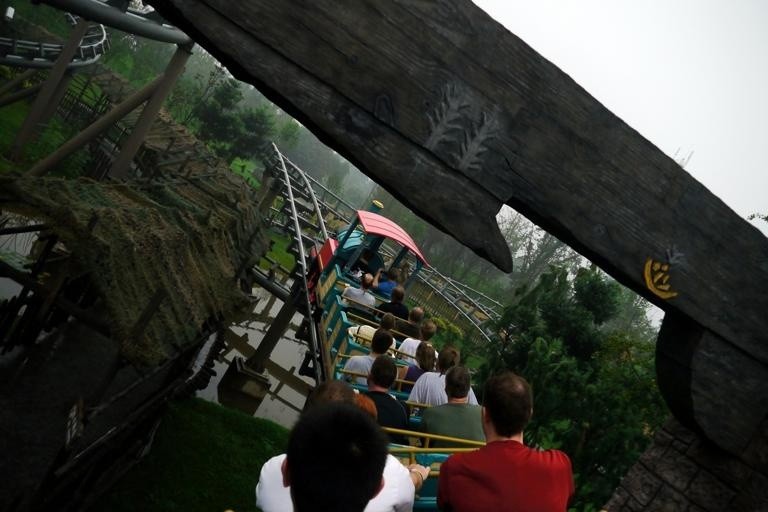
[409,468,425,481]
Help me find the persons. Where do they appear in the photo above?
[438,370,577,511]
[342,264,486,448]
[281,397,389,511]
[254,381,432,511]
